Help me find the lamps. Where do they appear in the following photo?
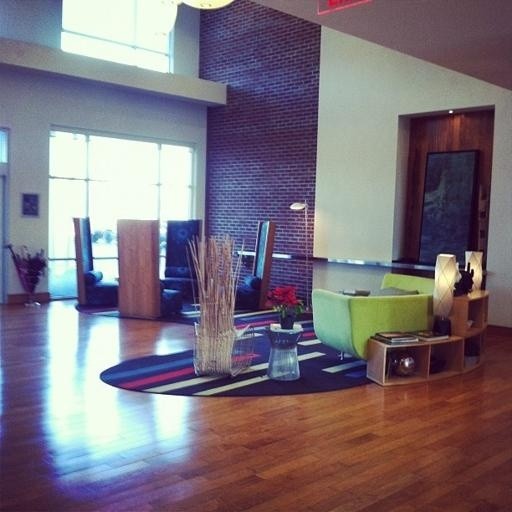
[74,217,118,306]
[234,221,274,312]
[164,220,202,298]
[116,219,183,319]
[312,272,438,362]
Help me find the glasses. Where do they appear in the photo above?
[432,254,458,337]
[290,200,312,314]
[464,249,484,292]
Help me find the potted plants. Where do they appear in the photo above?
[416,149,479,266]
[21,193,39,216]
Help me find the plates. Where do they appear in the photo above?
[366,337,463,386]
[449,294,490,373]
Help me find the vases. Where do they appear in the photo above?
[263,287,305,317]
[4,243,48,285]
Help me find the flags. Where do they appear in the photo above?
[279,315,295,329]
[20,274,41,307]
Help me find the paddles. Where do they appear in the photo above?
[93,302,312,336]
[99,334,372,396]
